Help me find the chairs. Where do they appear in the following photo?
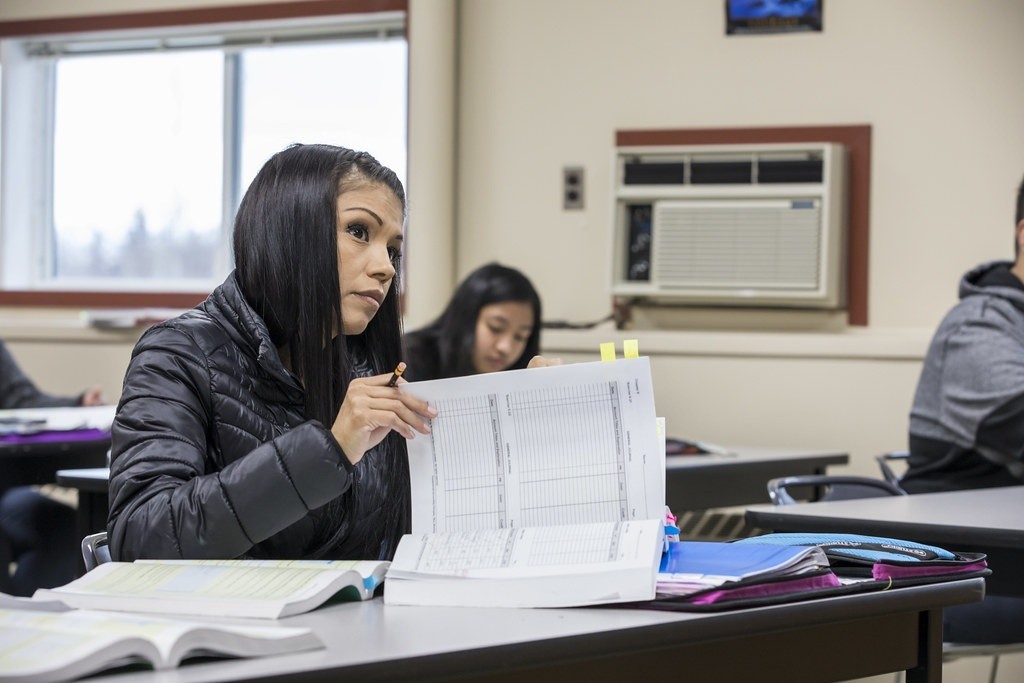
[768,476,903,507]
[81,532,113,574]
[876,451,1024,683]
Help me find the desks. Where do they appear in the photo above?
[0,404,120,494]
[745,482,1024,598]
[665,450,853,541]
[0,532,988,683]
[56,469,112,543]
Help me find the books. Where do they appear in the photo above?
[383,355,680,609]
[33,558,392,619]
[0,609,326,683]
[657,540,829,595]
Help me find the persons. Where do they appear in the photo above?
[0,339,105,596]
[897,179,1024,645]
[106,144,567,561]
[401,263,542,382]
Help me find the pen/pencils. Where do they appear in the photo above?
[384,362,407,387]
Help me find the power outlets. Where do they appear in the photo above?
[564,167,583,210]
